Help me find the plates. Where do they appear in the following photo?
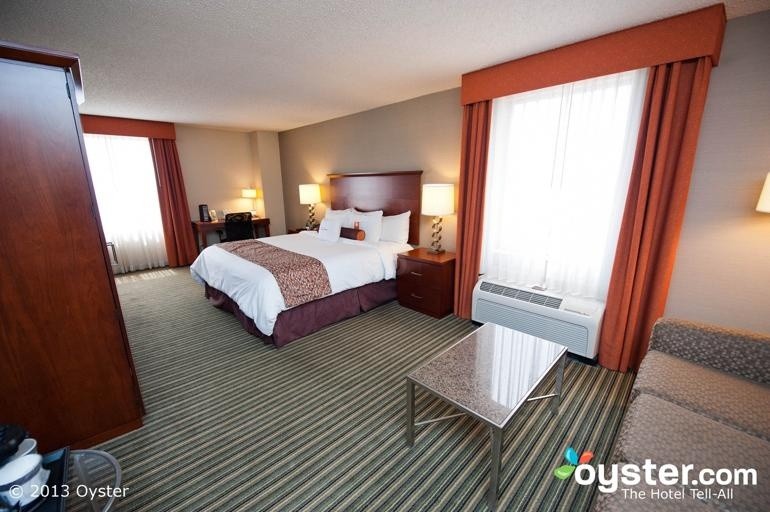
[0,443,70,512]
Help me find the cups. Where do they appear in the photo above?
[0,438,39,470]
[0,453,48,512]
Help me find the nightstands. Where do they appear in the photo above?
[396,248,456,319]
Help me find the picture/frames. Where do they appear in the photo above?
[209,210,217,221]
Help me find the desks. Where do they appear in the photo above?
[192,216,270,255]
[65,449,122,512]
[406,322,568,512]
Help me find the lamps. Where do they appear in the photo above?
[755,171,770,213]
[299,184,320,229]
[242,189,256,217]
[422,184,454,255]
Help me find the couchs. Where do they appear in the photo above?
[588,317,770,512]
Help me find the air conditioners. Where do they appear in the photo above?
[471,277,606,366]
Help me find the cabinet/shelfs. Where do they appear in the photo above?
[0,43,147,456]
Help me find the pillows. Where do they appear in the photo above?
[318,218,341,242]
[349,209,383,242]
[380,210,411,243]
[339,227,365,240]
[325,208,353,227]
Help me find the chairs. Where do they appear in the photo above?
[216,212,255,242]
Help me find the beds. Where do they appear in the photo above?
[202,170,423,346]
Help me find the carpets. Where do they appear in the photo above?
[61,265,639,512]
